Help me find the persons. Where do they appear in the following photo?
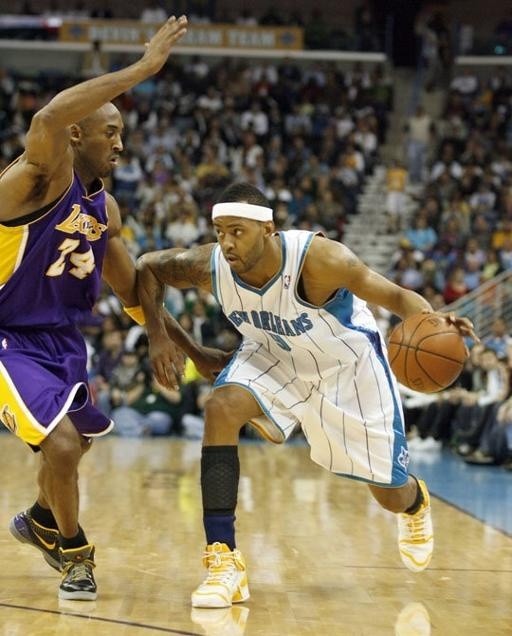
[0,13,189,600]
[135,184,482,607]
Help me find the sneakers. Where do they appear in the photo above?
[191,606,250,636]
[58,543,101,602]
[189,545,253,614]
[395,602,431,636]
[7,504,66,574]
[396,480,438,575]
[410,432,512,472]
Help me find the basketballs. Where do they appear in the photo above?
[387,314,466,393]
